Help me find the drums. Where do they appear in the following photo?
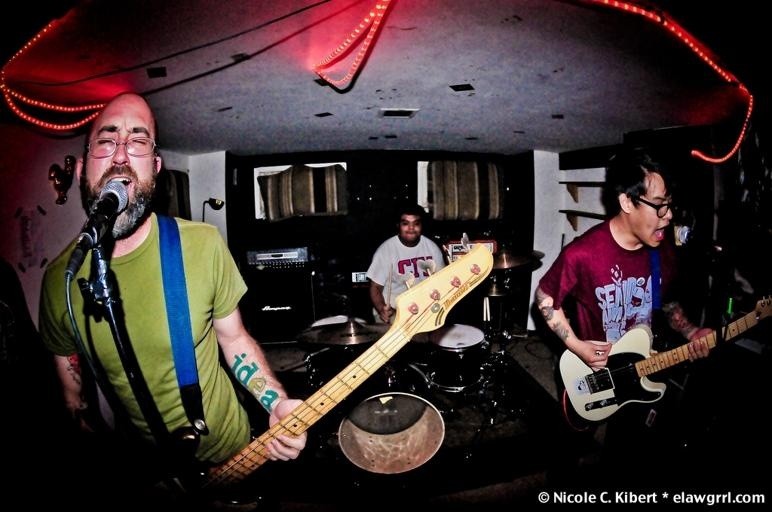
[304,346,351,393]
[428,322,488,395]
[336,364,448,475]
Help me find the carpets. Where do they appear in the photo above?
[241,353,567,505]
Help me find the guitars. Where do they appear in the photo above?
[70,232,494,512]
[558,292,772,423]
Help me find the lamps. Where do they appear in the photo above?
[202,198,224,222]
[49,155,76,205]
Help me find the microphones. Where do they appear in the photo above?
[65,181,129,280]
[674,225,725,273]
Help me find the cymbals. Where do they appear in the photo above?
[475,247,545,298]
[296,319,392,346]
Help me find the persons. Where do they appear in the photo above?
[522,142,713,511]
[33,88,313,510]
[357,201,453,345]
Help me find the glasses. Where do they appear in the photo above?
[637,197,675,217]
[88,138,157,159]
